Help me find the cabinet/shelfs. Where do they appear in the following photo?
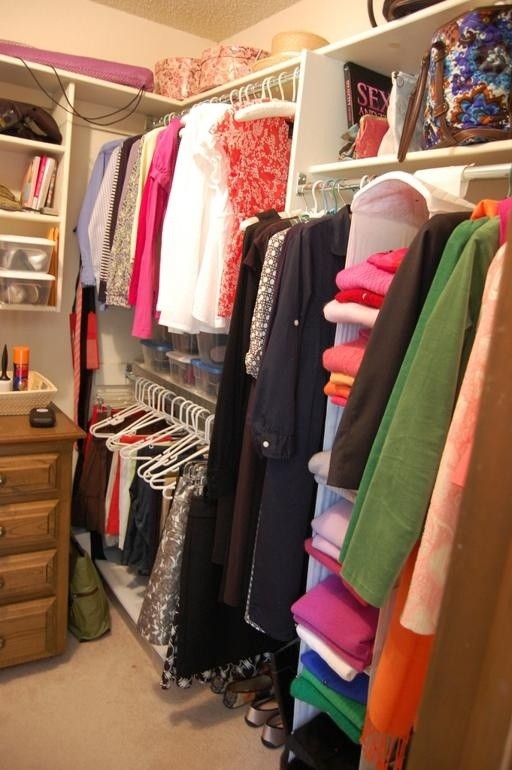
[288,170,476,770]
[0,402,88,669]
[0,54,75,312]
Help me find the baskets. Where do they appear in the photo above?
[0,369,59,415]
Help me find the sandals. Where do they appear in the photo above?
[207,668,288,749]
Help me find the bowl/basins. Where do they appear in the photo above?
[270,31,329,54]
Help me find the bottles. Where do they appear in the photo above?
[12,347,30,391]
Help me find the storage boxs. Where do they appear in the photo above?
[0,234,56,274]
[0,274,55,305]
[139,319,228,402]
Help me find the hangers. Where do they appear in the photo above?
[153,67,299,123]
[296,174,378,219]
[89,378,215,500]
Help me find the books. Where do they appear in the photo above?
[344,61,391,130]
[18,153,57,214]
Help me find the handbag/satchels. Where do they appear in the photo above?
[392,1,512,168]
[68,528,112,644]
